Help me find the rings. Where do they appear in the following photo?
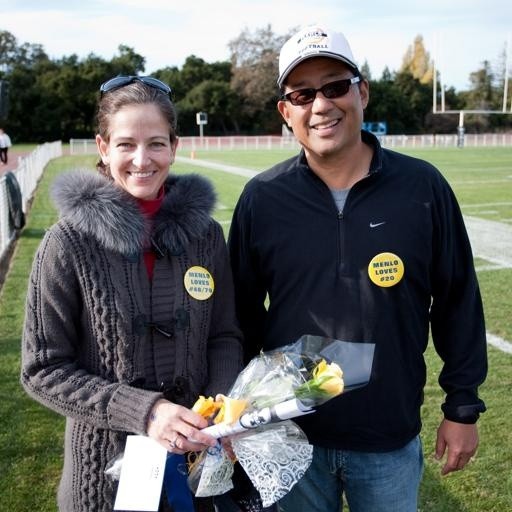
[169,436,179,448]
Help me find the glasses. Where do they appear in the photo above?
[277,76,363,106]
[100,76,172,101]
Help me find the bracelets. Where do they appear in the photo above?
[441,399,486,416]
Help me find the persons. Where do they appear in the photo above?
[227,24,488,511]
[19,78,245,512]
[0,128,12,165]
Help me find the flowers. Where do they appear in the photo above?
[295,356,345,405]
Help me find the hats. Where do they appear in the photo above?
[277,25,357,89]
